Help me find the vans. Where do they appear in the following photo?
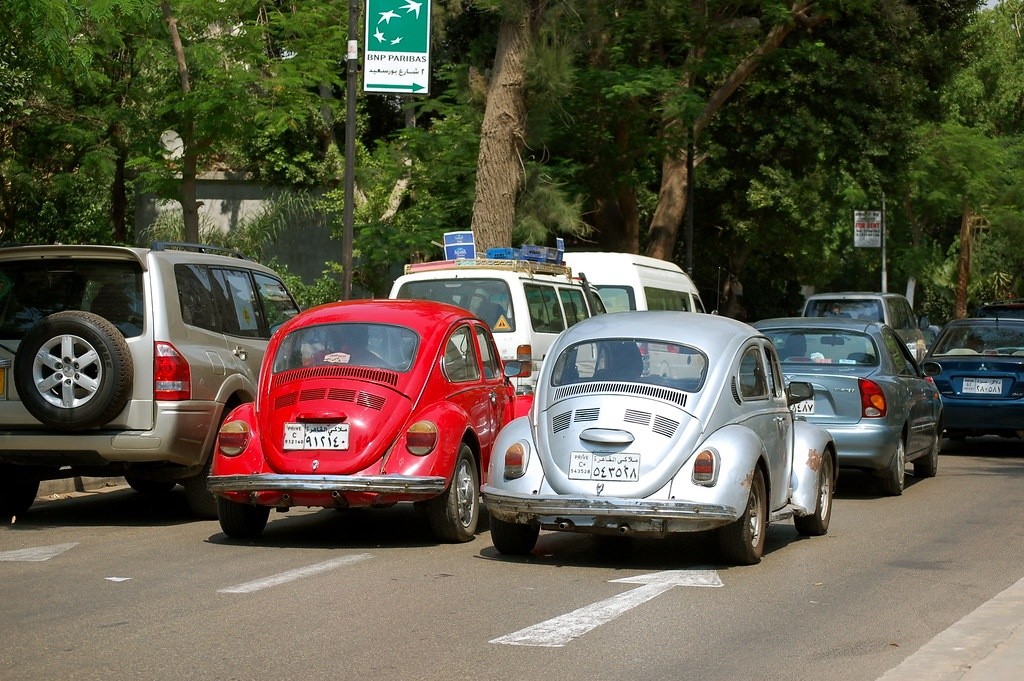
[559,251,720,380]
[378,238,608,395]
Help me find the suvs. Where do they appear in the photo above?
[945,296,1024,350]
[0,240,334,521]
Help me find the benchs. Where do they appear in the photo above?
[775,347,876,363]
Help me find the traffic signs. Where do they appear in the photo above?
[362,0,431,96]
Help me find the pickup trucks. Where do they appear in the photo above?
[768,290,929,365]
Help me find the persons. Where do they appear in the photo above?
[475,302,507,320]
[615,342,664,384]
[341,325,374,361]
[787,332,808,356]
[91,284,141,335]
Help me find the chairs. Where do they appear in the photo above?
[551,302,581,329]
[475,303,513,329]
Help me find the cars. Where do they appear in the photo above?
[716,320,944,498]
[205,300,535,545]
[918,317,1024,441]
[480,308,841,566]
